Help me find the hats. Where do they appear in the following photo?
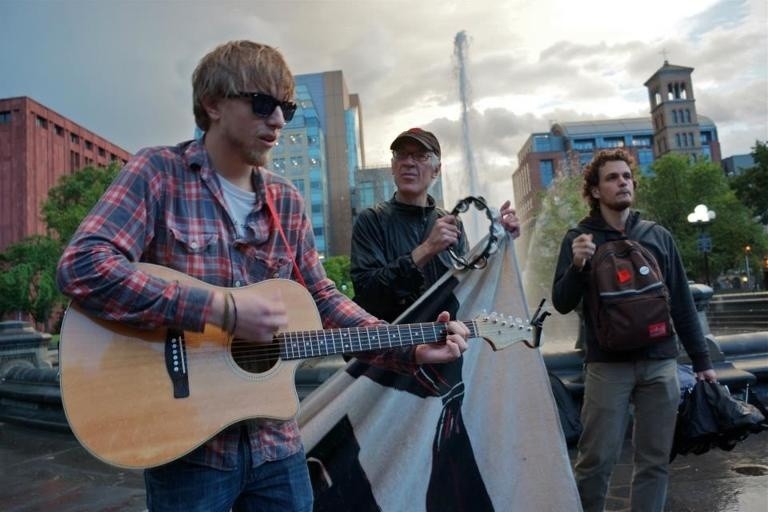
[390,128,441,158]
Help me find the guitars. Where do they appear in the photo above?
[58,265,540,471]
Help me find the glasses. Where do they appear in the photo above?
[393,151,433,161]
[221,92,298,123]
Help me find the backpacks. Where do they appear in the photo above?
[567,220,671,363]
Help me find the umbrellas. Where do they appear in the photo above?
[668,376,768,466]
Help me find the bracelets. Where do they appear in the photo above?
[220,291,238,336]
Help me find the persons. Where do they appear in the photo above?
[354,125,523,322]
[58,38,471,512]
[551,149,715,512]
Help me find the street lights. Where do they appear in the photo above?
[744,243,752,289]
[687,204,716,286]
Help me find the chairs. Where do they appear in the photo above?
[706,335,757,417]
